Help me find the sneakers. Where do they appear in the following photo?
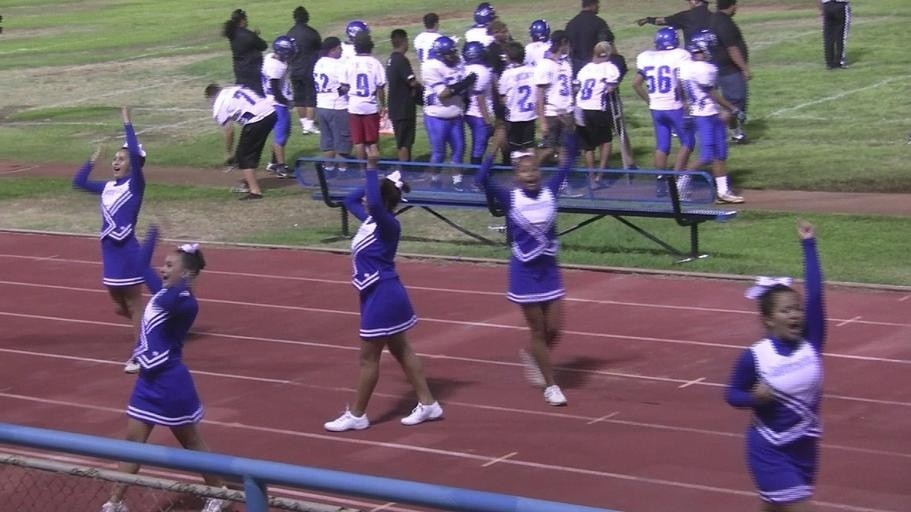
[200,485,235,512]
[543,385,567,406]
[100,500,128,512]
[123,361,141,373]
[400,401,444,427]
[714,190,745,204]
[322,410,370,432]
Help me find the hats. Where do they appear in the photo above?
[592,41,612,64]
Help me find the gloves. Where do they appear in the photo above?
[731,108,748,122]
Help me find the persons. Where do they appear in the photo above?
[288,5,323,136]
[474,107,577,405]
[222,8,268,101]
[311,0,628,198]
[72,104,146,375]
[819,0,852,71]
[102,221,234,512]
[322,143,444,432]
[205,82,276,202]
[632,0,753,205]
[725,218,826,511]
[260,34,299,180]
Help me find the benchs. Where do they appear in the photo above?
[294,155,738,265]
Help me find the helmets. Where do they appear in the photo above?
[272,35,298,61]
[654,26,679,50]
[462,41,488,64]
[685,26,720,61]
[529,19,550,41]
[346,20,368,43]
[474,2,495,24]
[432,36,458,67]
